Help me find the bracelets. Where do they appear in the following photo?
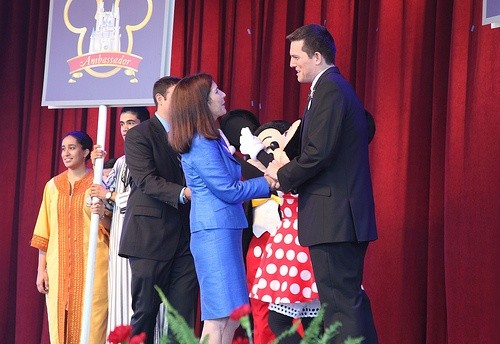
[182,187,189,203]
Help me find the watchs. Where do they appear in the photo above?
[106,190,114,205]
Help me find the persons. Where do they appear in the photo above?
[91,107,162,344]
[170,73,279,344]
[29,131,111,344]
[120,75,198,344]
[274,23,379,344]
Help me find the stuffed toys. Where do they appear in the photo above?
[218,105,378,344]
